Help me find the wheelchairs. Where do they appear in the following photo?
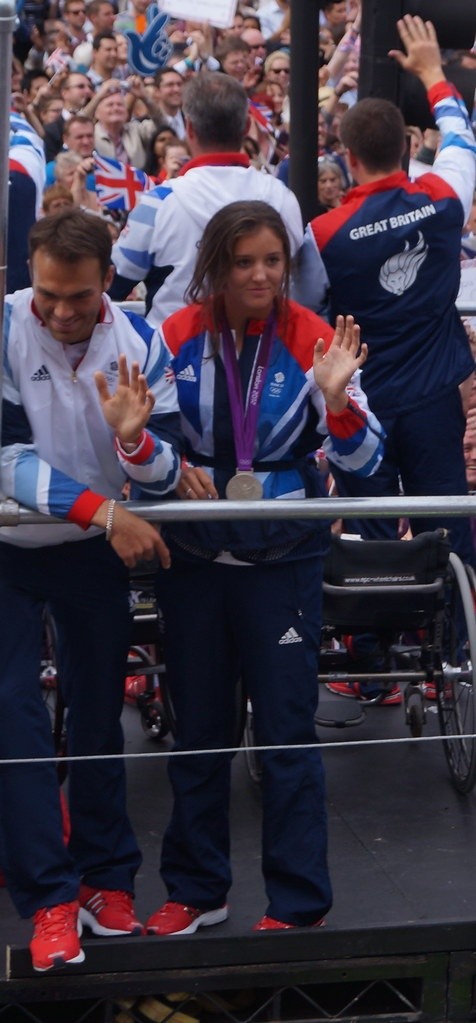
[40,578,246,788]
[241,526,476,794]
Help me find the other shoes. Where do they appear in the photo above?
[60,788,72,846]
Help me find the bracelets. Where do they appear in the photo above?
[106,498,116,540]
[118,432,144,447]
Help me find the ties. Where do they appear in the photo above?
[171,118,178,131]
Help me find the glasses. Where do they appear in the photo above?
[67,84,93,89]
[68,10,86,15]
[252,43,269,49]
[271,68,290,74]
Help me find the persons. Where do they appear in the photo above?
[9,0,295,704]
[106,72,328,336]
[316,0,475,258]
[131,201,385,936]
[297,14,475,707]
[0,208,179,969]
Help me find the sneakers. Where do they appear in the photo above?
[146,901,229,934]
[411,678,452,700]
[326,682,401,704]
[30,898,85,971]
[252,916,326,930]
[125,675,163,707]
[78,882,140,936]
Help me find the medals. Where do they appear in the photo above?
[226,473,263,500]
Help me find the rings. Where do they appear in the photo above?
[186,488,192,495]
